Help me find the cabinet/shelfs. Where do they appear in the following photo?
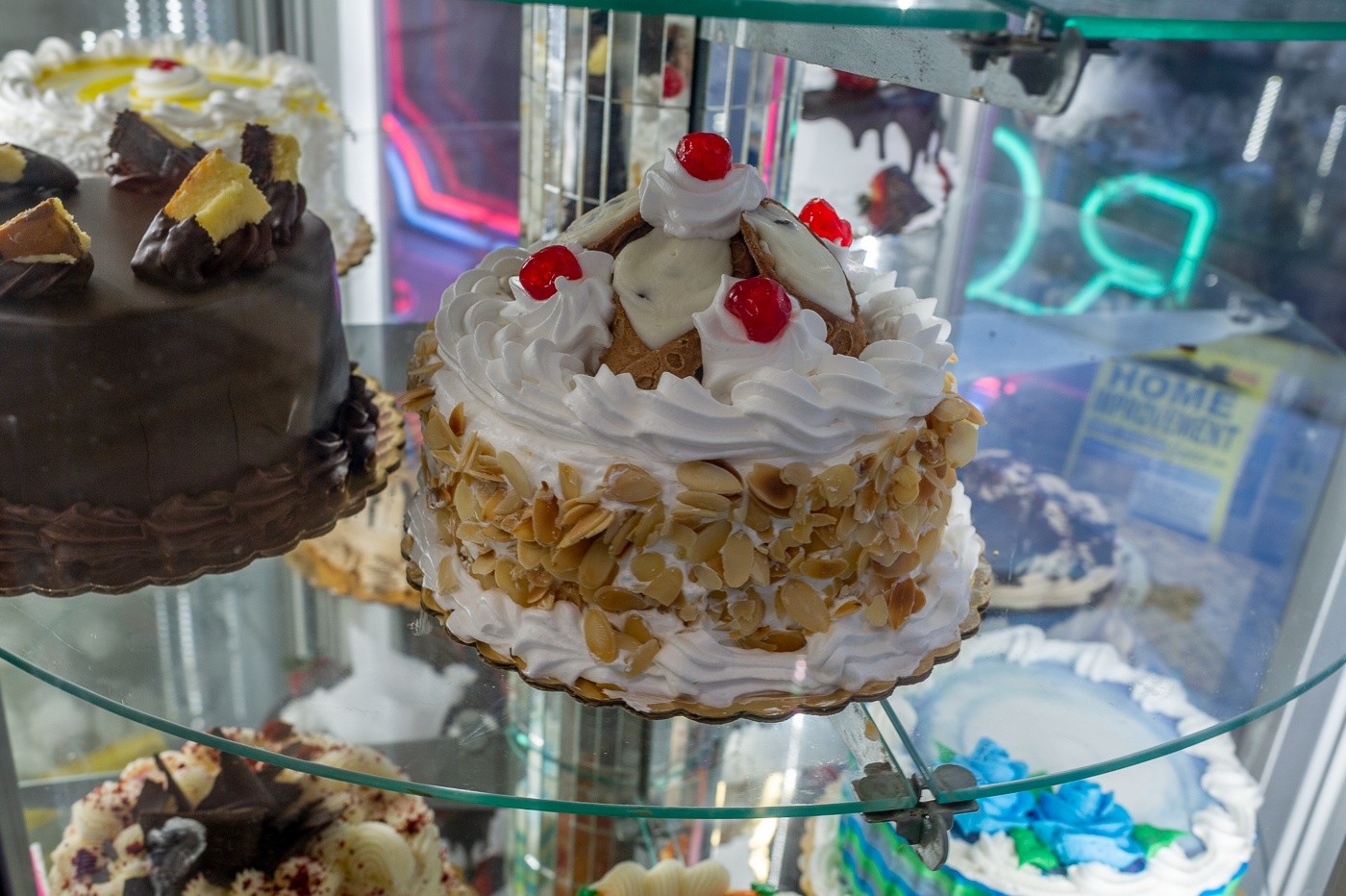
[0,0,1346,896]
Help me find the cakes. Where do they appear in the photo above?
[807,626,1263,895]
[1,107,380,591]
[41,404,784,896]
[0,30,357,266]
[947,446,1126,643]
[581,11,952,234]
[404,133,987,704]
